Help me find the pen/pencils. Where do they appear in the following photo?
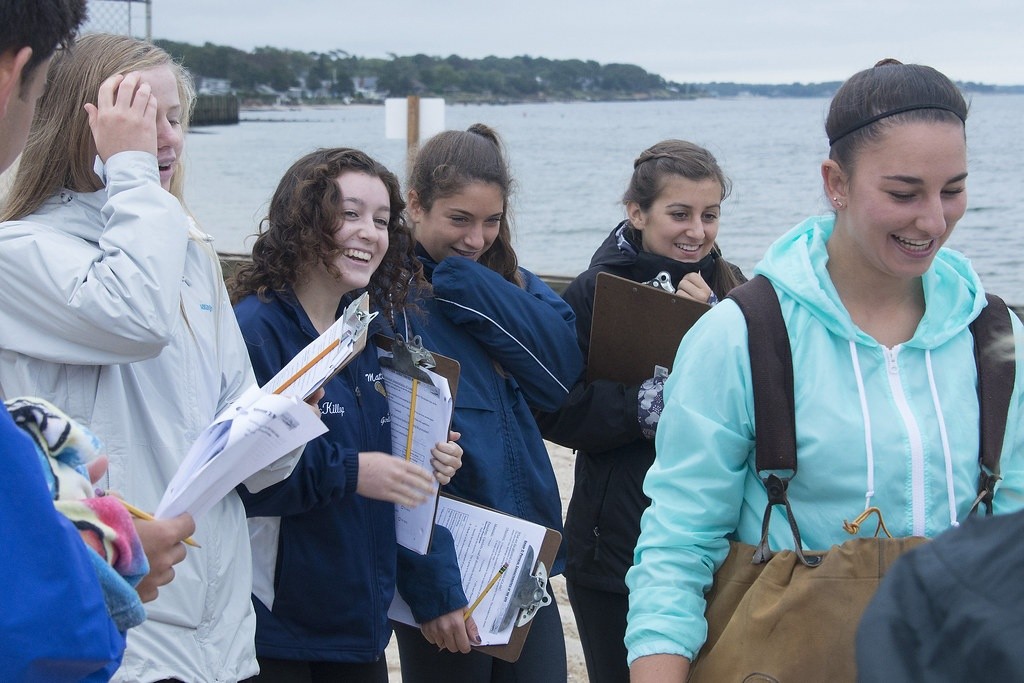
[405,379,418,462]
[439,562,510,652]
[273,329,352,394]
[95,489,202,548]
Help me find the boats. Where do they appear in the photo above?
[355,89,386,105]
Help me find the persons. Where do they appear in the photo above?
[0,1,329,683]
[849,510,1024,682]
[623,59,1023,681]
[533,140,749,683]
[231,124,586,683]
